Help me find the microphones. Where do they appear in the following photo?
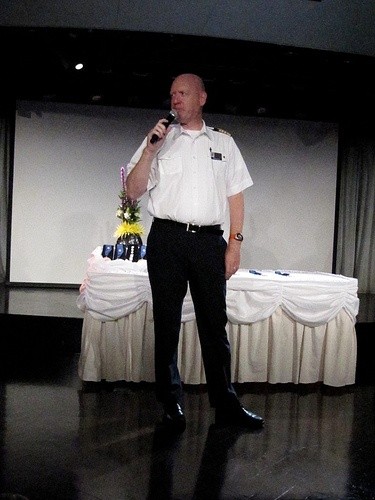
[150,109,177,144]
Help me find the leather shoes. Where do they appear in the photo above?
[162,400,185,422]
[215,406,265,428]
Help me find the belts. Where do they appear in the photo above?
[153,217,223,236]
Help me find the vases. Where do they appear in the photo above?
[117,234,143,262]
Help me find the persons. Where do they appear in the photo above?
[124,74,263,434]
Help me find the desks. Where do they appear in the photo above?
[77,246,360,392]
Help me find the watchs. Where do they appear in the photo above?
[229,233,244,242]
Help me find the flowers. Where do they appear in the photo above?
[113,165,146,240]
